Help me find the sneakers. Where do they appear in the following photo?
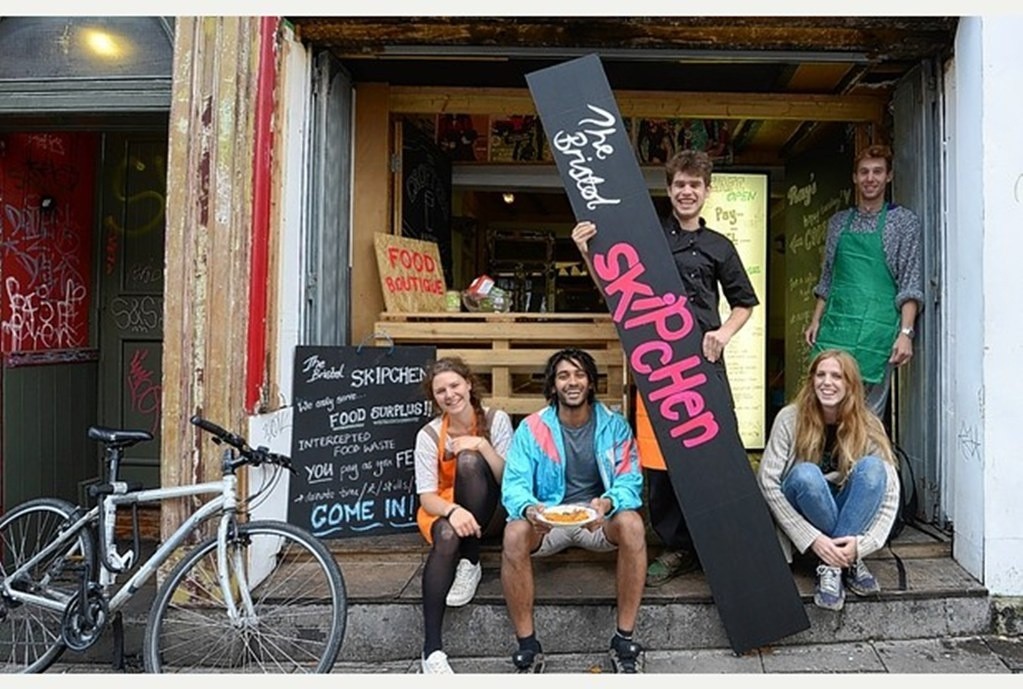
[446,560,483,607]
[842,559,880,597]
[814,565,845,611]
[420,649,455,675]
[644,551,695,586]
[512,641,545,674]
[608,636,642,673]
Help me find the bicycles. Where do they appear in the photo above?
[0,414,348,673]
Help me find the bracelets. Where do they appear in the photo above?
[474,436,483,448]
[446,505,462,521]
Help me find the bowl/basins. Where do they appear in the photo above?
[463,291,513,313]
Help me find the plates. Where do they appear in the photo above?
[536,505,598,527]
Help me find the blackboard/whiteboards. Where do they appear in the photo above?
[286,345,440,540]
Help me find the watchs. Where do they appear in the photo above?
[901,328,915,339]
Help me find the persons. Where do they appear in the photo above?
[803,144,924,423]
[414,357,514,674]
[758,349,901,612]
[501,350,649,674]
[570,149,760,588]
